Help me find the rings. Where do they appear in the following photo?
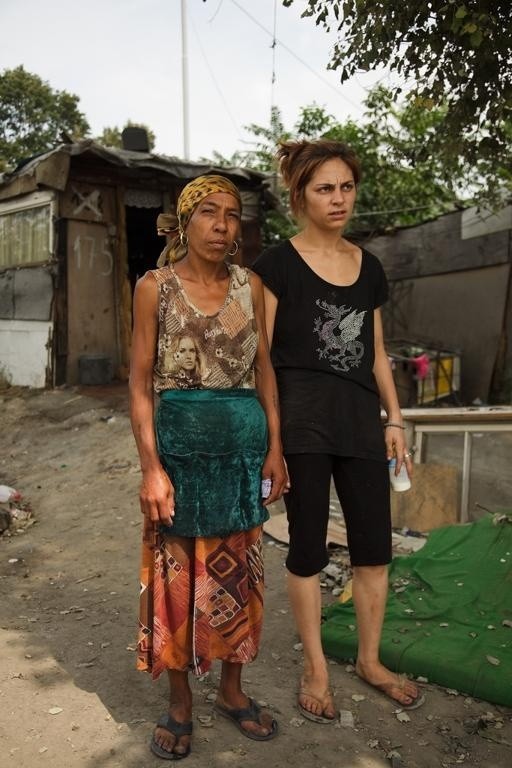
[403,453,410,458]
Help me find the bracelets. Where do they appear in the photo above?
[382,422,407,429]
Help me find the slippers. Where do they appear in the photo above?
[213,695,279,742]
[150,711,194,762]
[380,671,426,712]
[294,676,337,725]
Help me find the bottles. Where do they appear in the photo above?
[389,457,412,494]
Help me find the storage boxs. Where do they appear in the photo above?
[389,461,459,534]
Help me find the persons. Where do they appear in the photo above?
[129,175,290,766]
[164,331,203,384]
[253,136,426,720]
